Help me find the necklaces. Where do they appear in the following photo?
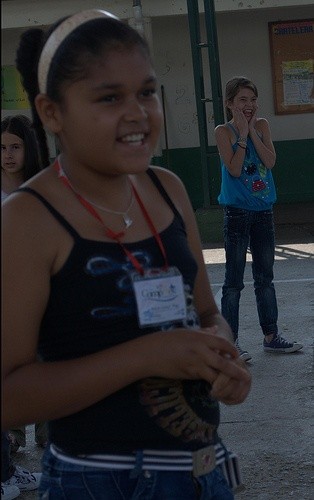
[57,153,137,231]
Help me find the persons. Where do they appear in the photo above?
[0,115,42,204]
[0,10,254,500]
[0,426,43,500]
[212,75,303,365]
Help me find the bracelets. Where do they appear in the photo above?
[236,135,250,158]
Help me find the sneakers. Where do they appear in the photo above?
[0,482,20,500]
[263,332,304,353]
[234,338,252,361]
[0,468,42,491]
